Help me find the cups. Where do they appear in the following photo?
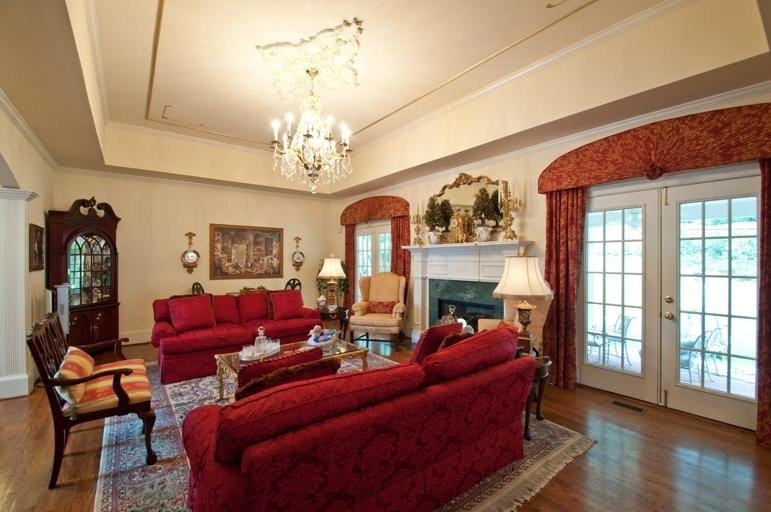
[269,337,280,354]
[242,344,254,359]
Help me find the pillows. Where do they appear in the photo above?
[367,300,395,313]
[54,345,96,405]
[153,291,304,333]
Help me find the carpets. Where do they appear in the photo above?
[93,353,598,512]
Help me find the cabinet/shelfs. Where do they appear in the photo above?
[47,197,120,354]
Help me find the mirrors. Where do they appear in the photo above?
[433,173,508,243]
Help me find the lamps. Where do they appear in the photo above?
[317,258,346,312]
[256,20,363,193]
[492,246,554,357]
[408,201,425,245]
[498,176,522,240]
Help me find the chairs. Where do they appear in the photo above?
[349,272,406,351]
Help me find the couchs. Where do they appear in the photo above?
[152,291,323,385]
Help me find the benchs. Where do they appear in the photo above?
[27,312,156,489]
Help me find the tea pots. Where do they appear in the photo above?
[254,327,269,356]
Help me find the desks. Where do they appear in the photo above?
[316,307,350,341]
[524,355,552,441]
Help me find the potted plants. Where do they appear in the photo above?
[491,190,505,241]
[441,200,454,241]
[473,188,495,242]
[425,196,444,244]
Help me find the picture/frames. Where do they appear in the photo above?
[210,224,284,280]
[29,223,44,272]
